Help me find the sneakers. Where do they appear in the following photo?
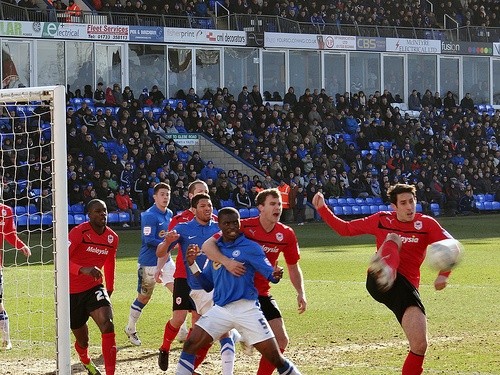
[83,359,102,375]
[125,328,141,346]
[178,331,188,343]
[1,341,12,351]
[158,349,169,371]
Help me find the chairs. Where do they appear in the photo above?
[0,97,500,232]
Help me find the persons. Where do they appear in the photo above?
[312,183,457,375]
[0,1,500,375]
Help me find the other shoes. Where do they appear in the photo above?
[298,223,304,225]
[123,223,129,227]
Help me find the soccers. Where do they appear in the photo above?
[426,239,464,271]
